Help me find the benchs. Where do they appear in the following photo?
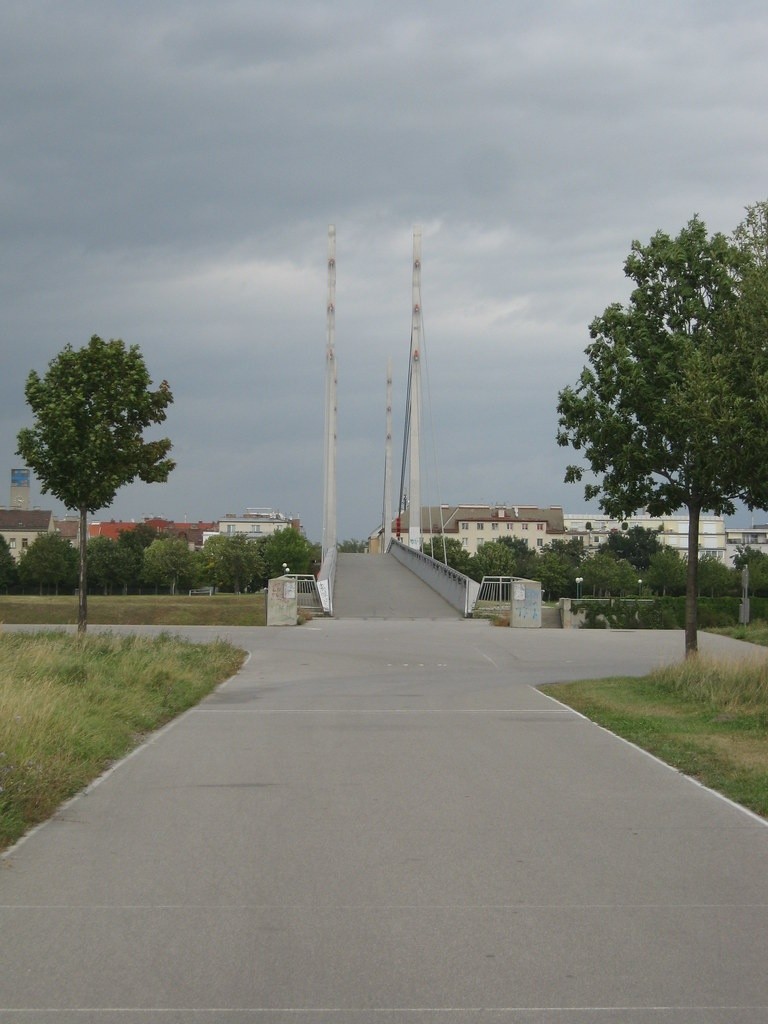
[189,589,212,596]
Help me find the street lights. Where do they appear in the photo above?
[576,577,583,599]
[638,579,642,599]
[283,563,290,575]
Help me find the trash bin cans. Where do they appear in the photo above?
[75,589,80,596]
[739,598,749,624]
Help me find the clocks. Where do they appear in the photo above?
[14,495,25,505]
